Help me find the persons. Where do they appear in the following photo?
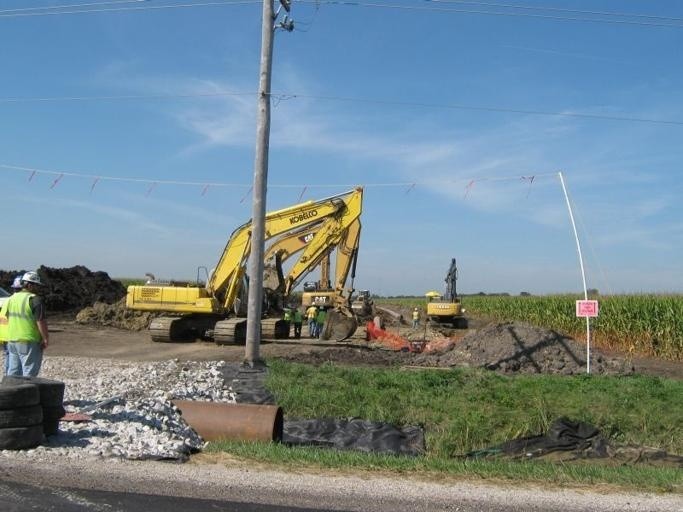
[304,301,317,337]
[283,309,292,321]
[0,275,23,376]
[0,270,48,378]
[292,308,302,339]
[373,310,381,331]
[410,307,421,329]
[312,307,327,338]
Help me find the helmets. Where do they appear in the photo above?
[11,275,24,289]
[319,306,324,310]
[414,307,418,311]
[22,270,47,287]
[312,301,315,305]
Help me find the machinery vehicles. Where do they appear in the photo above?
[425,290,464,329]
[125,187,364,344]
